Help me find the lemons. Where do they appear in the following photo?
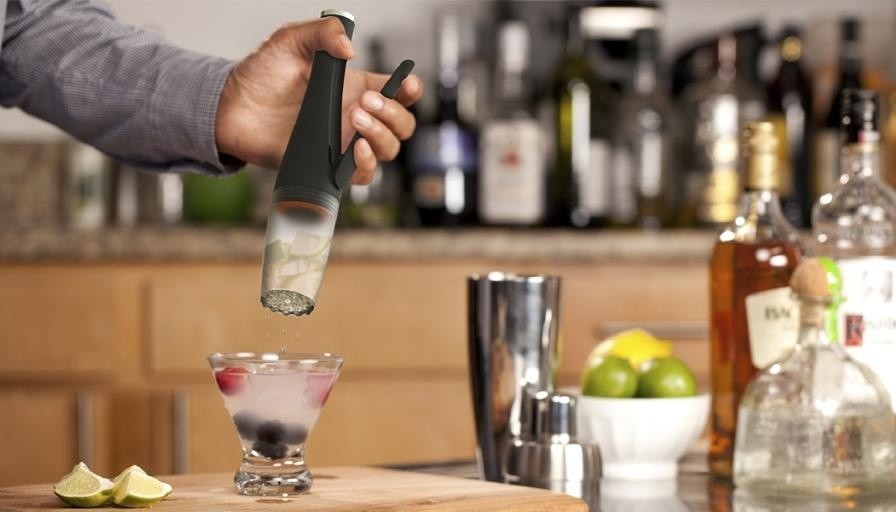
[579,328,698,398]
[52,461,173,507]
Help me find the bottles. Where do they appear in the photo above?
[708,89,895,510]
[410,0,865,228]
[504,386,603,510]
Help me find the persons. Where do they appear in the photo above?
[0,0,423,186]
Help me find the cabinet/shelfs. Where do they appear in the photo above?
[1,229,896,486]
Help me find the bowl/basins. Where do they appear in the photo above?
[562,387,712,500]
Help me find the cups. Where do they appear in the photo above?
[465,272,561,484]
[208,351,344,498]
